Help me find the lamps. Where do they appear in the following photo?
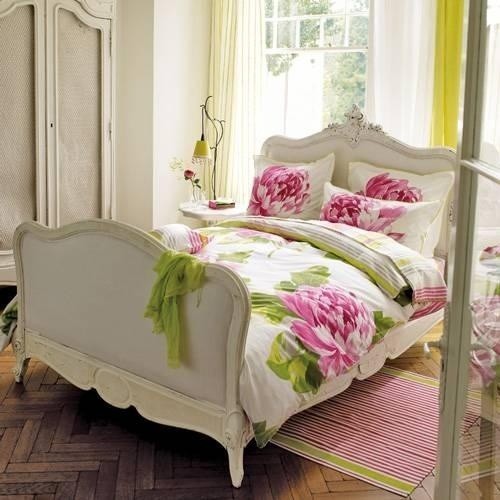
[192,96,226,200]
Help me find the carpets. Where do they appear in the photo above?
[267,365,481,499]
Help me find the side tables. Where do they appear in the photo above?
[178,199,250,228]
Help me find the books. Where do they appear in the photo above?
[209,199,236,209]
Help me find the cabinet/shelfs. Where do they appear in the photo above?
[0,0,115,285]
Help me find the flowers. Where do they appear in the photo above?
[184,170,201,201]
[353,172,422,203]
[323,194,406,240]
[249,264,396,395]
[247,165,310,217]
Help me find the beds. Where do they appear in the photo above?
[11,102,457,491]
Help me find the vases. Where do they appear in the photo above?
[192,188,201,203]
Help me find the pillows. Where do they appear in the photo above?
[319,181,442,250]
[245,153,336,219]
[147,223,215,253]
[347,161,455,204]
